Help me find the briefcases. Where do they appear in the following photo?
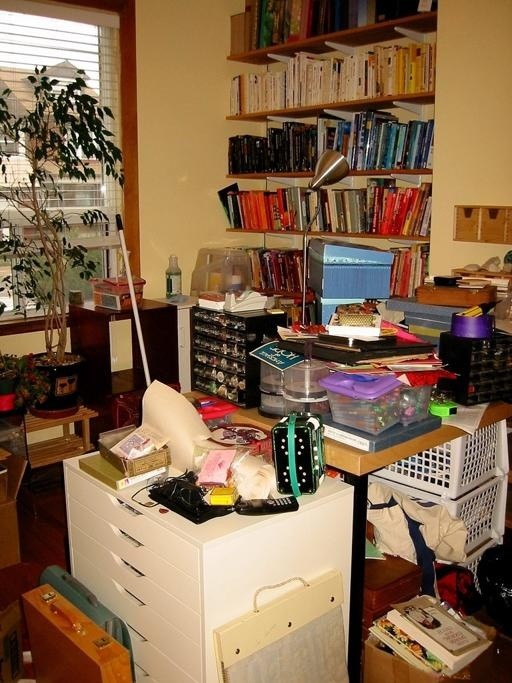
[22,582,133,682]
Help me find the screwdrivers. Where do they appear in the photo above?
[457,299,502,316]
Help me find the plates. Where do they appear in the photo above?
[210,426,267,444]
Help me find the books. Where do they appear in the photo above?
[368,595,492,678]
[327,314,381,336]
[217,178,432,236]
[322,414,442,453]
[227,291,276,313]
[390,243,429,298]
[244,0,437,51]
[227,245,308,294]
[79,454,167,490]
[227,110,434,176]
[227,43,437,118]
[386,298,437,314]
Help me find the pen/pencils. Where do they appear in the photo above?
[330,389,403,434]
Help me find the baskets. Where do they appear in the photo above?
[338,309,375,327]
[371,420,510,499]
[367,474,507,569]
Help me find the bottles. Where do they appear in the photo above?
[164,255,182,299]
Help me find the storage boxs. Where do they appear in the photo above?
[316,371,434,437]
[305,238,395,328]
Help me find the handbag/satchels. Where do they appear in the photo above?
[39,564,131,650]
[366,483,468,563]
[272,412,326,498]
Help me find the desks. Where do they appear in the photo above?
[228,400,512,683]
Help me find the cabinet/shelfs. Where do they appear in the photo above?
[63,451,355,682]
[220,1,438,298]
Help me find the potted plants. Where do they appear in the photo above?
[0,59,123,421]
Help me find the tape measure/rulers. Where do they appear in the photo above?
[430,399,458,416]
